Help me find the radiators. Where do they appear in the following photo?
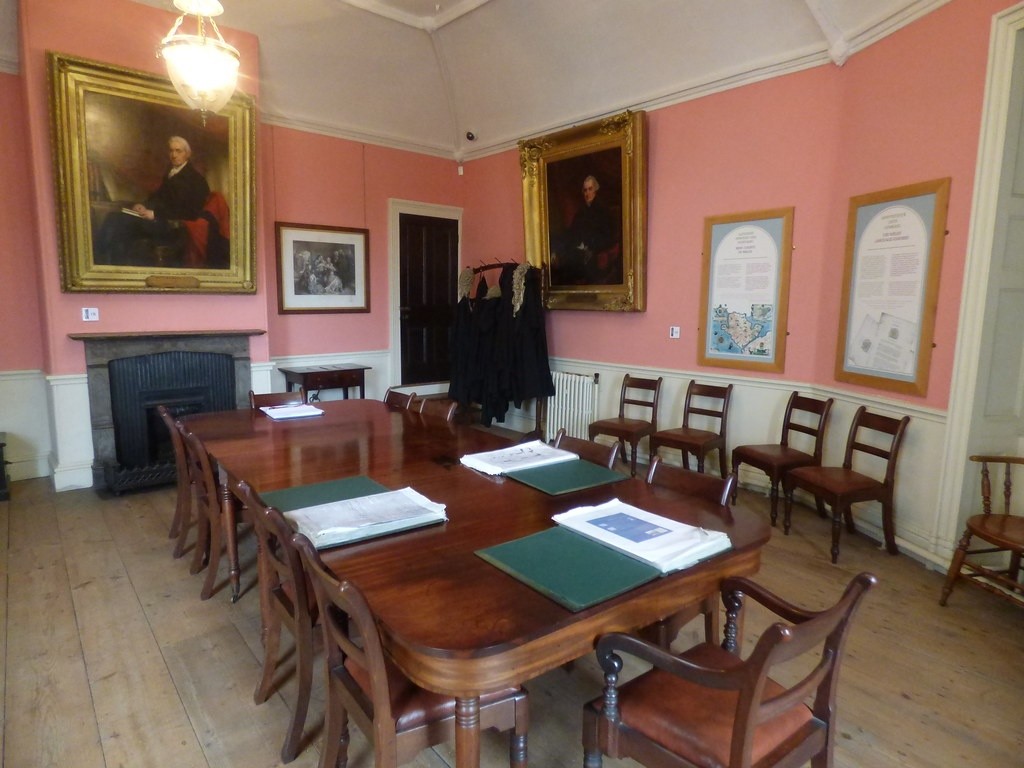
[546,370,599,443]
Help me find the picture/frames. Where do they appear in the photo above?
[833,177,951,400]
[696,205,795,373]
[274,221,371,315]
[517,109,649,313]
[45,52,257,297]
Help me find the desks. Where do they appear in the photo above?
[278,362,373,403]
[182,398,772,768]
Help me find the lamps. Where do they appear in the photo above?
[155,0,241,127]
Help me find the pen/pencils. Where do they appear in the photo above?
[268,405,296,409]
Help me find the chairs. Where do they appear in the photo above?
[589,373,664,478]
[732,391,834,527]
[157,404,194,559]
[291,533,532,768]
[235,481,361,762]
[384,387,417,409]
[553,427,622,469]
[420,396,457,423]
[646,456,738,652]
[782,406,911,564]
[176,421,227,602]
[649,380,733,480]
[580,571,876,768]
[249,387,306,409]
[939,455,1024,609]
